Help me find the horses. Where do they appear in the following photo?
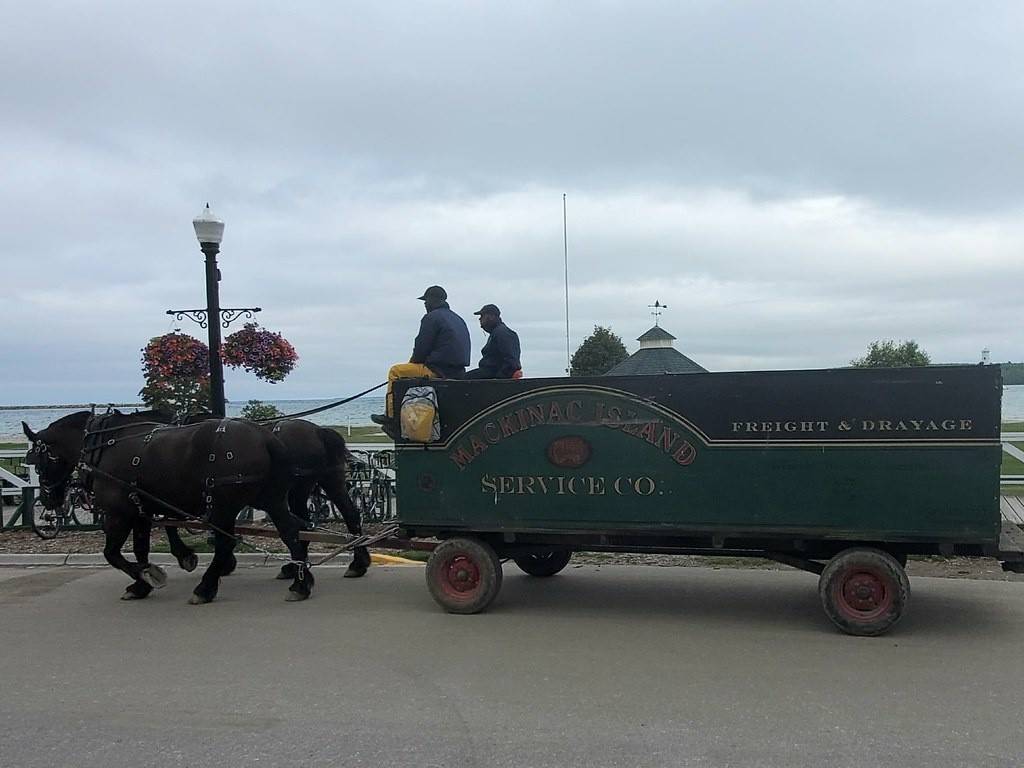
[21,403,373,606]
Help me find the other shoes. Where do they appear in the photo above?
[381,426,395,440]
[371,414,394,432]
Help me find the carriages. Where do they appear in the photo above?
[19,363,1023,636]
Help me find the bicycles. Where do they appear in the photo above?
[30,463,105,540]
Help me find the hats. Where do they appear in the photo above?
[416,285,447,300]
[474,304,501,316]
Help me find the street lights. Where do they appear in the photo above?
[192,203,226,419]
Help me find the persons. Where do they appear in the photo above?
[370,284,471,440]
[466,304,523,381]
